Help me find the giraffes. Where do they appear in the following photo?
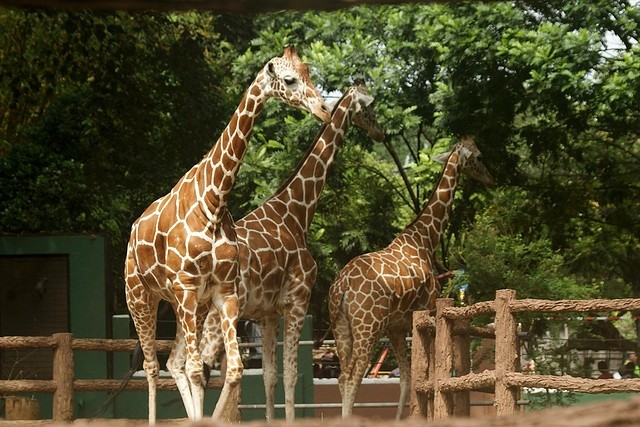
[313,133,496,421]
[123,42,334,427]
[198,75,385,422]
